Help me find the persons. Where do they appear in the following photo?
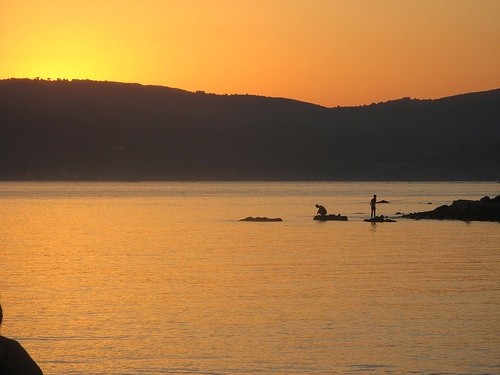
[370,194,377,218]
[315,204,327,216]
[0,304,45,375]
